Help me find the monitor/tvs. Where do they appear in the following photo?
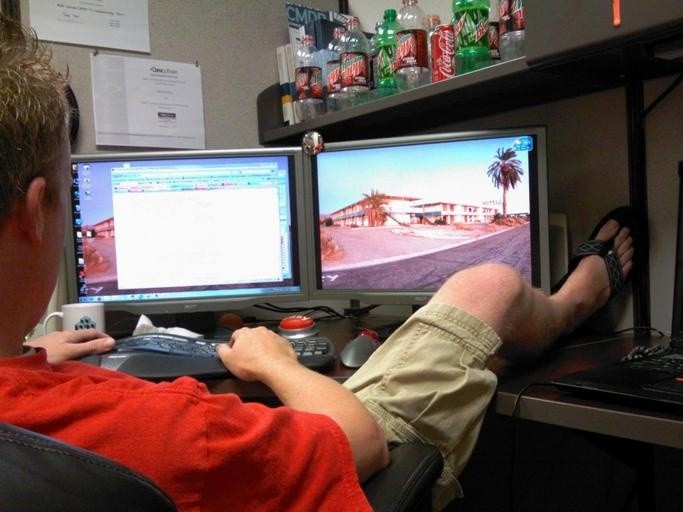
[65,147,309,341]
[302,126,551,340]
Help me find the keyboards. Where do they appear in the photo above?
[72,332,337,382]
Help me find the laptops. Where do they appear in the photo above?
[546,159,683,413]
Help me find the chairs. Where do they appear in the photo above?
[0,423,445,512]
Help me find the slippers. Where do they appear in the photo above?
[551,206,633,344]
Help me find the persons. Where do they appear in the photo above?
[0,17,635,512]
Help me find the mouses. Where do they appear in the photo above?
[339,328,382,368]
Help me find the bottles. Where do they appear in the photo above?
[293,0,525,121]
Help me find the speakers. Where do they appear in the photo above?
[548,213,569,289]
[25,247,69,340]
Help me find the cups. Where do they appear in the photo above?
[44,302,105,335]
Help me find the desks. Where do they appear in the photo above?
[204,0,683,451]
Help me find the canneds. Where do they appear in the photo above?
[431,24,456,83]
